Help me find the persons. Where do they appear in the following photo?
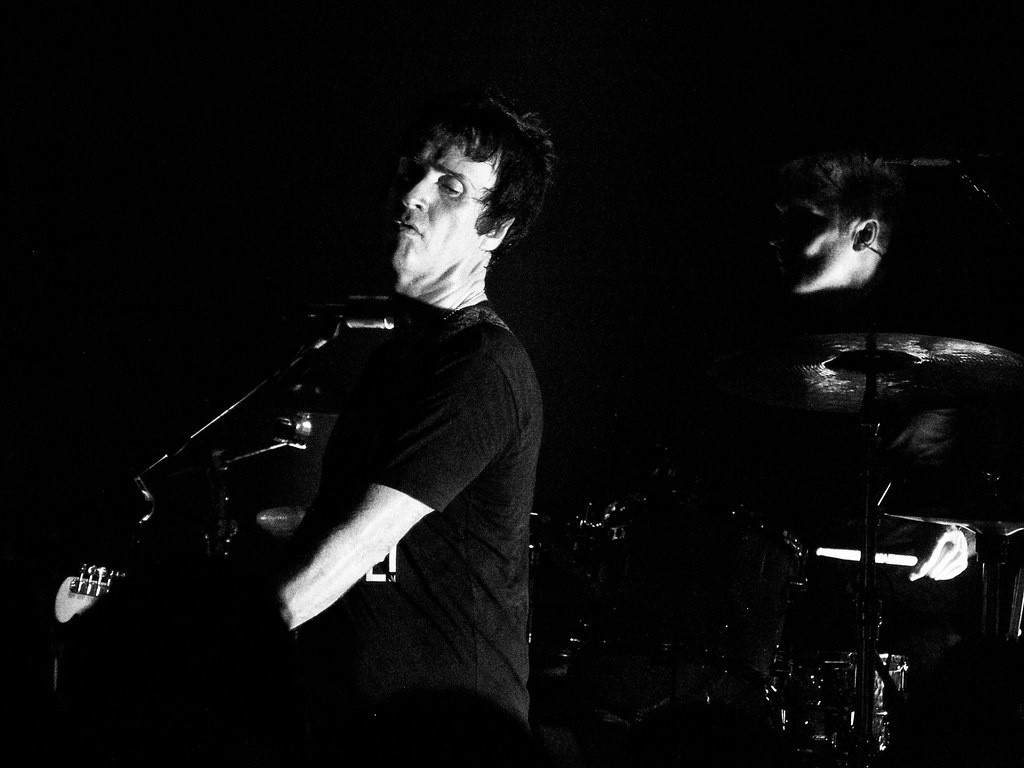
[769,154,1023,661]
[270,101,554,768]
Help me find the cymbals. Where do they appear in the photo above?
[707,332,1024,415]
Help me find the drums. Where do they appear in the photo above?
[529,486,806,706]
[754,644,911,768]
